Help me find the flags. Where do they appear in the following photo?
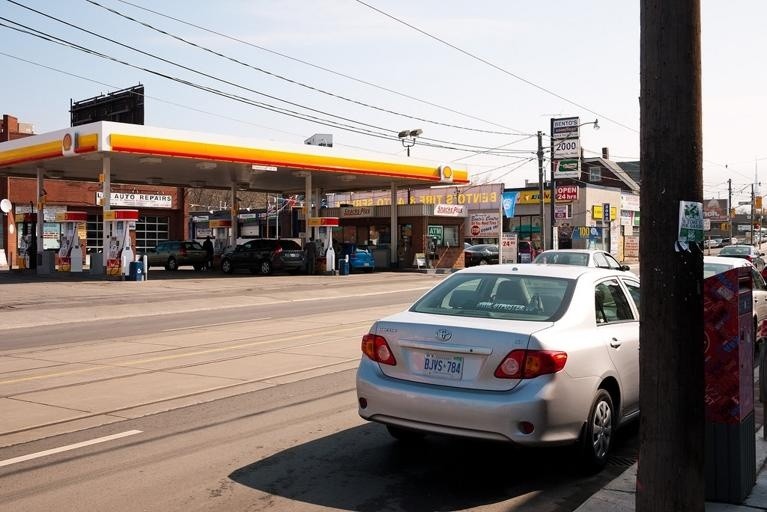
[500,192,517,219]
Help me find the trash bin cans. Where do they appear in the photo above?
[129,261,142,281]
[339,260,349,275]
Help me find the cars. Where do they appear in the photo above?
[137,241,212,273]
[333,241,377,273]
[703,227,766,360]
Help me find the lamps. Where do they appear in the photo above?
[46,170,64,179]
[449,289,480,311]
[144,177,161,185]
[236,182,249,192]
[335,174,357,182]
[139,157,162,167]
[529,294,561,316]
[195,162,217,171]
[495,278,532,305]
[189,180,209,188]
[290,170,311,179]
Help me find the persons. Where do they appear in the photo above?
[22,235,37,273]
[203,236,215,270]
[304,237,317,275]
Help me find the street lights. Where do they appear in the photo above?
[399,129,423,204]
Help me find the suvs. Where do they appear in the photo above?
[219,238,305,276]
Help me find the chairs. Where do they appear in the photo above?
[595,283,619,323]
